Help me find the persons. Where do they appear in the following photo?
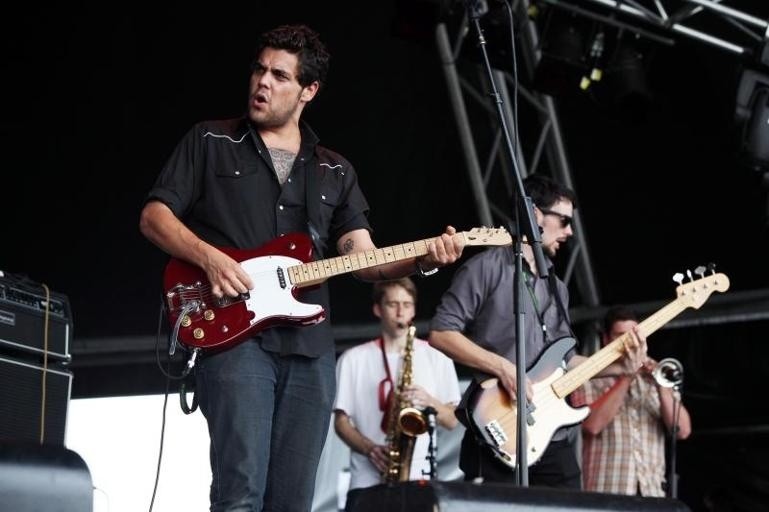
[565,304,693,498]
[138,26,465,511]
[332,274,464,511]
[431,170,650,492]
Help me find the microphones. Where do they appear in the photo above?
[665,368,682,381]
[423,404,442,435]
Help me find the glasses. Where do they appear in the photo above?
[543,210,575,229]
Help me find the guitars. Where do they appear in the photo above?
[466,262,732,473]
[160,223,514,355]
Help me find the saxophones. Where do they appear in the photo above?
[380,321,428,491]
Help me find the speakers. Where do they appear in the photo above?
[1,355,74,449]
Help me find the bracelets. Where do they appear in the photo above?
[414,255,443,279]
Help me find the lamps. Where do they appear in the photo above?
[574,43,615,90]
